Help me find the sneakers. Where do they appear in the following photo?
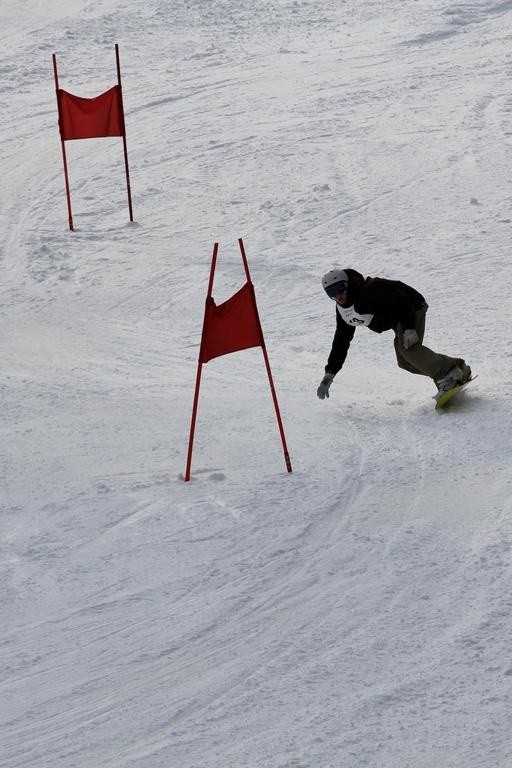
[433,360,472,402]
[321,268,349,290]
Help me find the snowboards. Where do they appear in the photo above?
[435,376,479,410]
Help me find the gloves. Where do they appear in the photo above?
[317,373,335,400]
[403,329,419,350]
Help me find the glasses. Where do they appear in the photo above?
[325,283,346,298]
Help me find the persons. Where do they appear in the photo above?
[317,268,471,402]
